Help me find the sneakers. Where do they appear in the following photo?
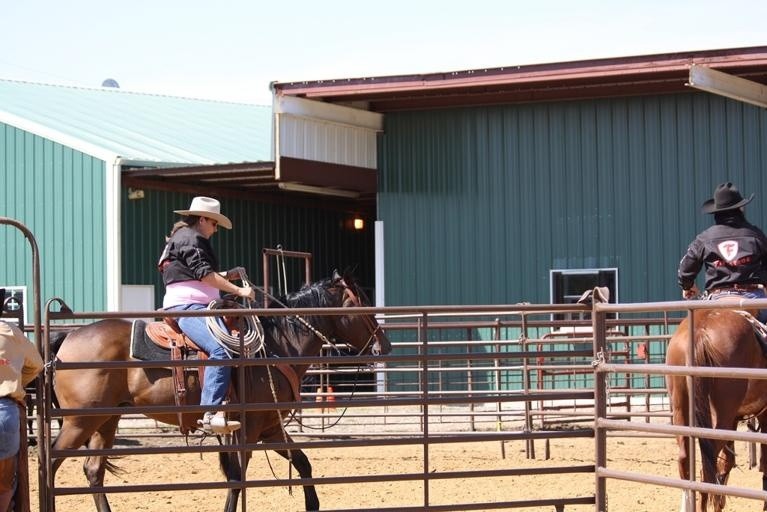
[203,411,241,432]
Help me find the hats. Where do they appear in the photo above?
[701,182,754,214]
[173,196,233,230]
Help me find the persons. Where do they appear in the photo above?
[159,195,256,432]
[678,183,767,324]
[0,320,44,461]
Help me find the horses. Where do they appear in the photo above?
[664,309,767,512]
[40,268,392,512]
